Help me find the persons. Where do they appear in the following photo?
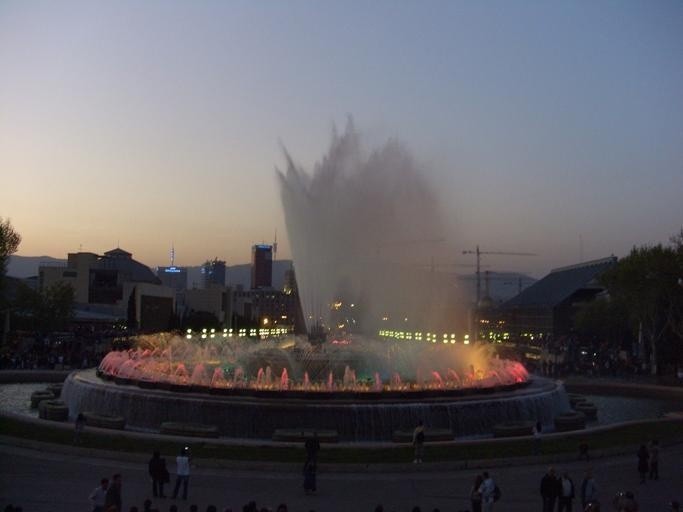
[302,454,320,496]
[303,438,321,462]
[410,419,429,463]
[148,449,167,498]
[168,448,192,501]
[87,479,109,511]
[106,474,121,512]
[468,439,660,512]
[529,422,544,455]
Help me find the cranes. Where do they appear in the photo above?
[416,238,543,303]
